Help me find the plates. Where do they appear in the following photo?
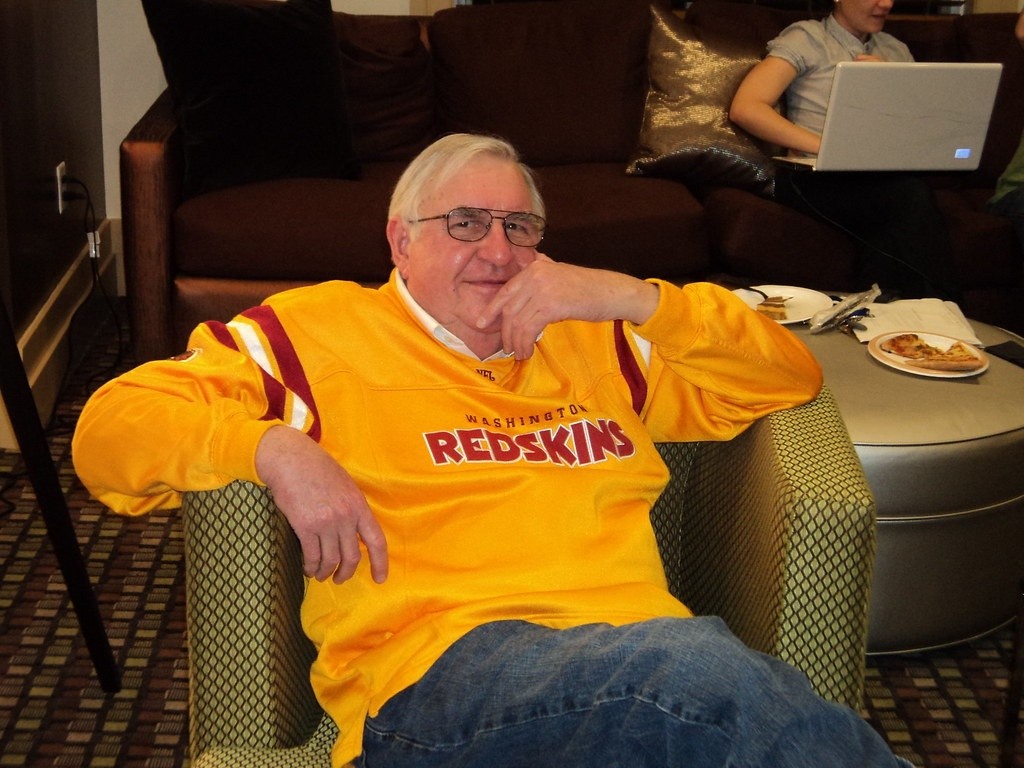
[731,285,833,324]
[868,331,990,378]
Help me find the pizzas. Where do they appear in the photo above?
[880,334,946,359]
[907,342,984,371]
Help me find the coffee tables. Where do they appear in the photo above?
[785,323,1024,663]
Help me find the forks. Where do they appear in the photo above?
[738,286,794,302]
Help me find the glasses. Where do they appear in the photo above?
[408,205,548,247]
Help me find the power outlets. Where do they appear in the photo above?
[57,161,70,214]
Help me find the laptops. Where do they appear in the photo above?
[771,62,1003,173]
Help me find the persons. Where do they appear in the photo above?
[72,132,919,768]
[730,0,980,314]
[988,11,1024,231]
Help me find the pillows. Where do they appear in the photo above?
[141,0,367,207]
[625,5,785,201]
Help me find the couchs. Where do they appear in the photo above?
[120,0,1024,367]
[182,382,877,768]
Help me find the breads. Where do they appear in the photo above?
[756,295,787,321]
